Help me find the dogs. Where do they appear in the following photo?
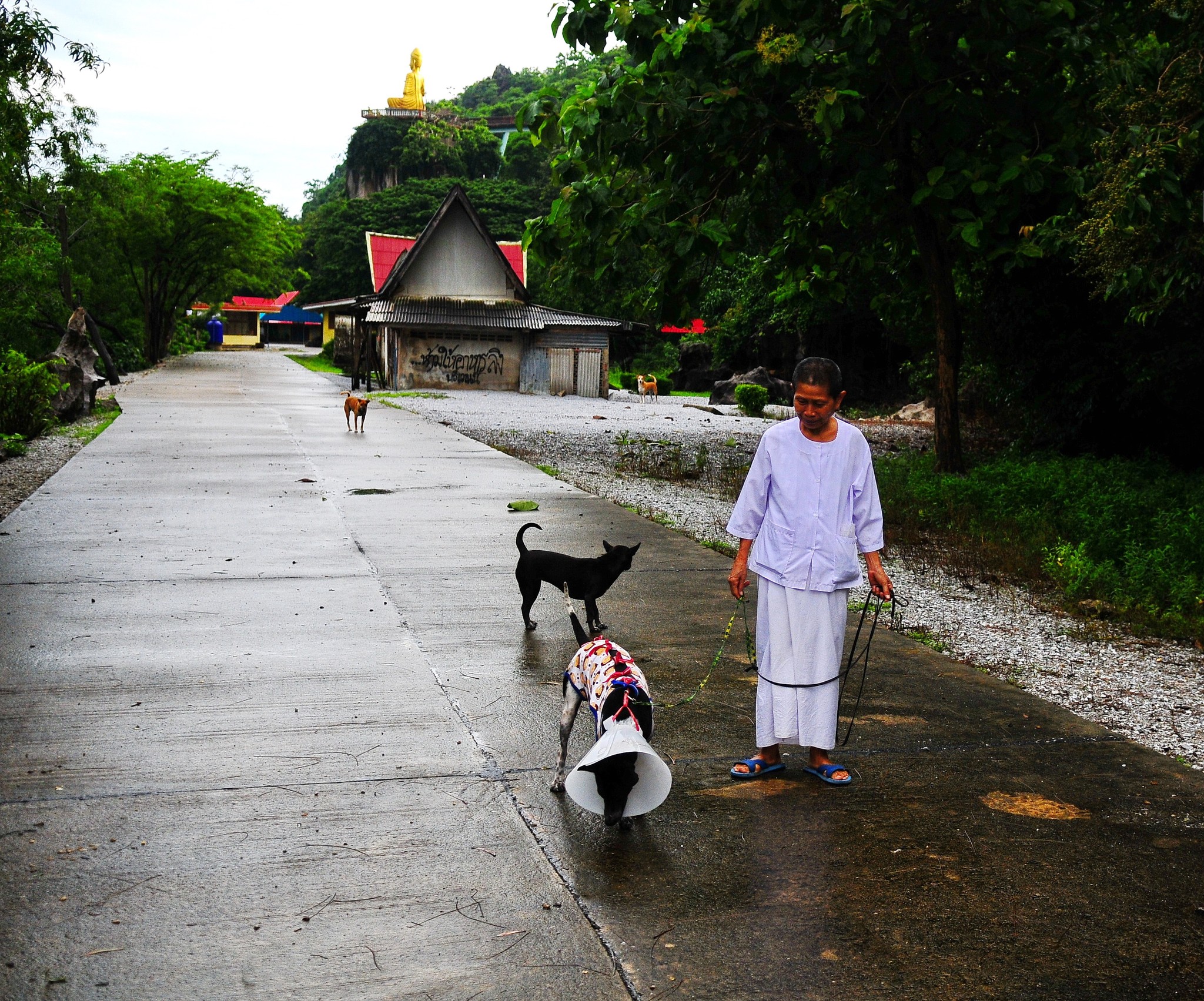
[547,576,675,830]
[635,373,659,404]
[510,520,642,636]
[339,390,371,433]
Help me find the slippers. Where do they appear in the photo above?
[731,757,785,776]
[803,763,851,783]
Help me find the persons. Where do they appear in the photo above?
[724,356,893,784]
